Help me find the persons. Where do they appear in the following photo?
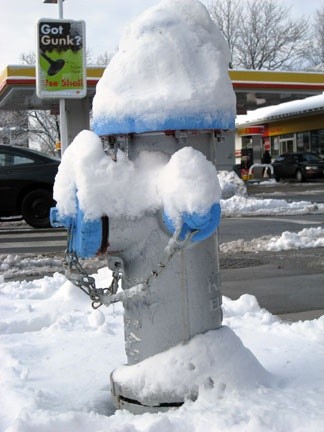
[260,150,272,176]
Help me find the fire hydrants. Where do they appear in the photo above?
[49,24,236,415]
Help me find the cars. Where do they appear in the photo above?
[0,143,61,229]
[272,151,324,183]
